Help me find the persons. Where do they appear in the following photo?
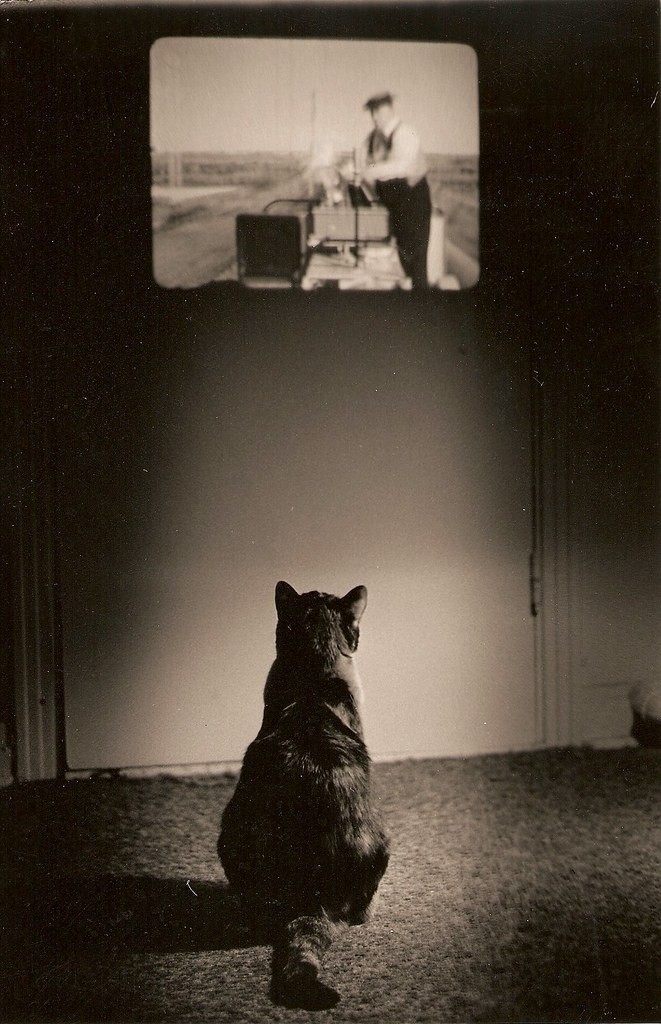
[342,92,431,289]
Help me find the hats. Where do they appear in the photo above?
[365,93,394,110]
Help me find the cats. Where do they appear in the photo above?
[216,581,391,1012]
